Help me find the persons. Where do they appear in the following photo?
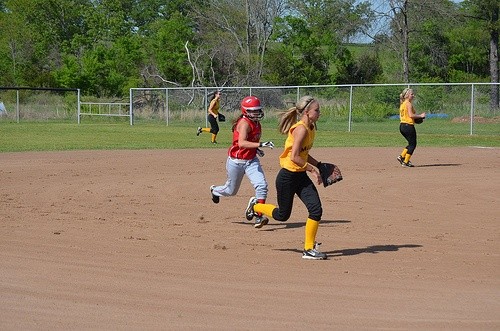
[396,88,426,167]
[196,90,225,145]
[209,95,270,229]
[245,97,328,260]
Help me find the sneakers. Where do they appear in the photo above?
[254,217,269,229]
[397,155,405,164]
[245,197,257,220]
[302,245,327,260]
[197,127,202,136]
[210,185,219,204]
[211,141,217,144]
[402,161,414,167]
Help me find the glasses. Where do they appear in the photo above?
[217,93,222,96]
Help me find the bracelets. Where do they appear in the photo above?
[302,163,307,169]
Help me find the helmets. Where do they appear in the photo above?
[241,96,264,115]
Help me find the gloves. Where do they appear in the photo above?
[260,142,275,149]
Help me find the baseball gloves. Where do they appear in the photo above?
[318,162,343,187]
[415,117,425,124]
[218,113,225,122]
